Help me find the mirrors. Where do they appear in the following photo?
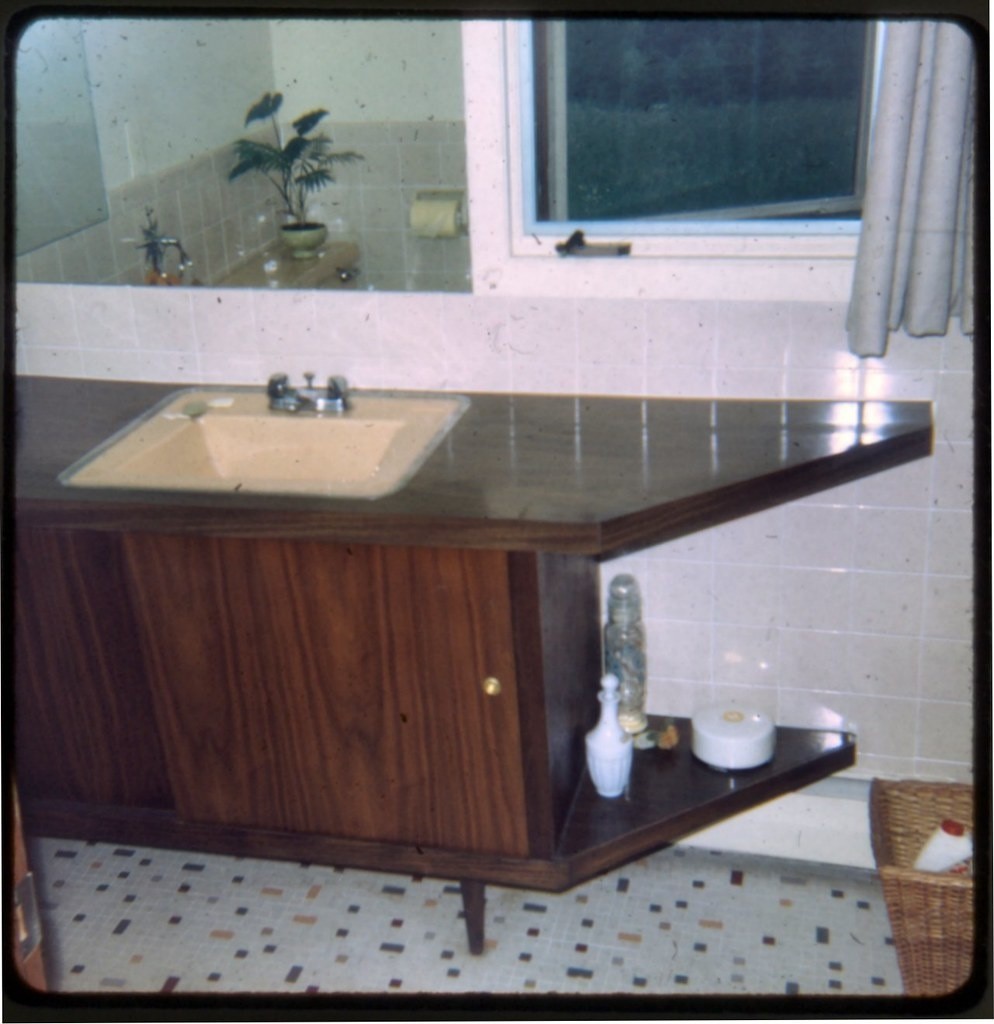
[12,19,881,298]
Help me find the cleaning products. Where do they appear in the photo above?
[909,819,970,879]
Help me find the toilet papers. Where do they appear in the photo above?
[408,198,461,240]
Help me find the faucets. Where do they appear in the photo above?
[272,371,330,422]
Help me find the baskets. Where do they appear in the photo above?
[867,777,972,996]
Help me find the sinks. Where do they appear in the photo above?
[55,377,478,505]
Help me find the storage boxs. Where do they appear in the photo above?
[868,769,973,999]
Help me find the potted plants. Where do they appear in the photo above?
[226,87,364,261]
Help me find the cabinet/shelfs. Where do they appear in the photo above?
[19,426,936,961]
